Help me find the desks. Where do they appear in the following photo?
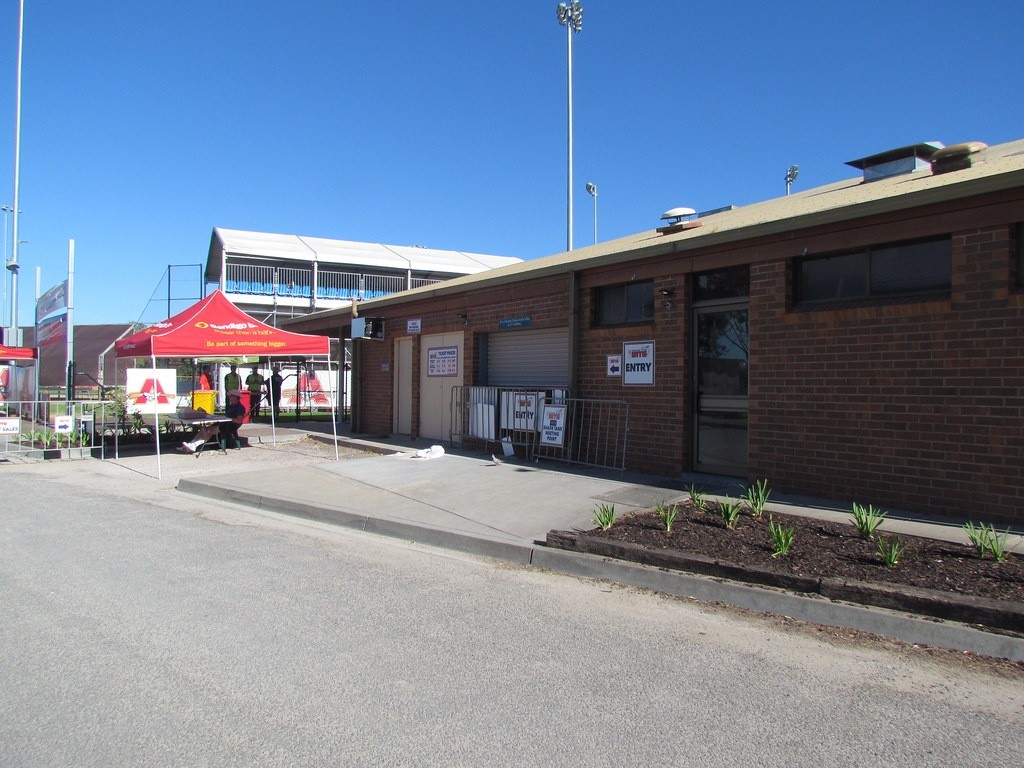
[182,416,231,458]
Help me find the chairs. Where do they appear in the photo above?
[222,422,241,449]
[225,279,395,302]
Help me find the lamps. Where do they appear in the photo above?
[456,313,465,319]
[660,289,670,297]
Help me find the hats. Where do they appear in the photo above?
[227,391,241,397]
[271,367,281,371]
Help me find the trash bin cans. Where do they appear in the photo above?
[189,390,218,417]
[227,390,251,424]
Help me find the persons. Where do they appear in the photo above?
[247,366,264,418]
[0,382,8,404]
[176,390,246,454]
[199,364,212,390]
[264,367,283,421]
[225,365,243,411]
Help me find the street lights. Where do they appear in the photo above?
[783,164,799,196]
[1,205,14,326]
[557,0,583,252]
[585,183,598,245]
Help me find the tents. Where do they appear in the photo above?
[0,346,40,422]
[115,289,339,480]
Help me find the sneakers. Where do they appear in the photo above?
[176,446,189,453]
[182,442,196,452]
